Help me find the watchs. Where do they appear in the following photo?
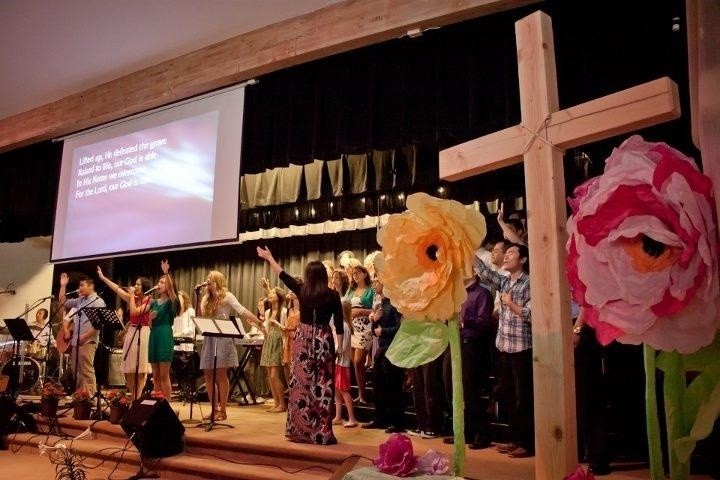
[573,325,582,334]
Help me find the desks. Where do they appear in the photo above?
[230,336,265,405]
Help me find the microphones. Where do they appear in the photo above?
[194,281,208,290]
[65,289,80,295]
[43,295,55,300]
[144,286,159,295]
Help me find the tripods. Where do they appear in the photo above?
[191,315,241,432]
[81,306,127,419]
[58,292,103,417]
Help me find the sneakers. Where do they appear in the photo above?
[407,429,421,435]
[421,431,440,438]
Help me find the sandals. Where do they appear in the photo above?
[344,421,358,427]
[215,411,227,421]
[204,410,219,419]
[332,419,342,425]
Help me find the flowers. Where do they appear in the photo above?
[372,192,488,480]
[565,134,716,480]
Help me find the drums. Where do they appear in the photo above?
[1,357,40,392]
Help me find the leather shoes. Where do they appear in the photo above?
[443,437,454,444]
[384,426,404,432]
[496,443,516,453]
[468,441,490,448]
[508,447,527,457]
[361,420,377,429]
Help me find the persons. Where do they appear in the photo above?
[571,289,599,391]
[257,246,344,445]
[199,271,262,420]
[322,260,335,290]
[407,360,440,438]
[149,260,182,403]
[331,268,349,297]
[337,250,354,269]
[343,257,362,278]
[63,308,77,341]
[496,201,528,246]
[247,287,288,413]
[97,265,152,399]
[476,241,510,277]
[472,243,531,458]
[283,299,300,394]
[331,300,358,427]
[344,265,375,406]
[172,289,196,354]
[361,274,405,433]
[259,276,295,306]
[28,309,57,347]
[58,272,106,398]
[443,270,491,449]
